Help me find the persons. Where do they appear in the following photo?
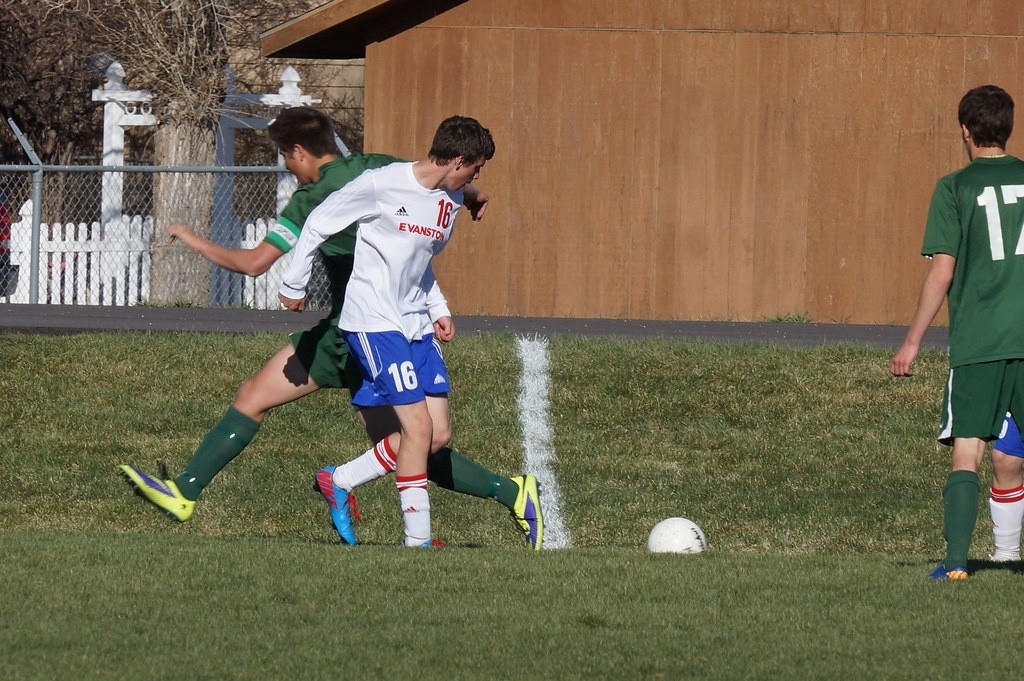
[888,85,1023,579]
[116,106,544,551]
[278,114,496,551]
[985,410,1024,562]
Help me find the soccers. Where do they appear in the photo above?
[649,517,706,554]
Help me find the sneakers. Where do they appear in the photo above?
[401,539,447,549]
[509,474,545,554]
[312,465,357,546]
[119,463,198,524]
[928,565,969,581]
